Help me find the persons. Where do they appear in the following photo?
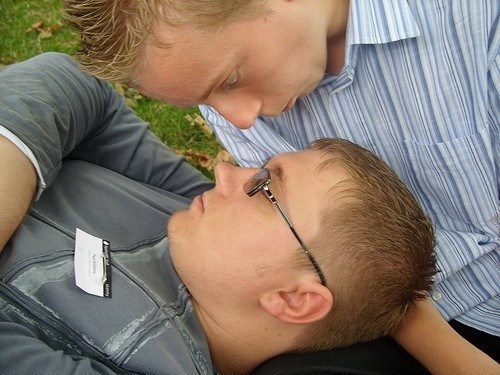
[56,1,500,374]
[1,49,442,375]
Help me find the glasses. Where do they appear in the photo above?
[243,168,325,287]
